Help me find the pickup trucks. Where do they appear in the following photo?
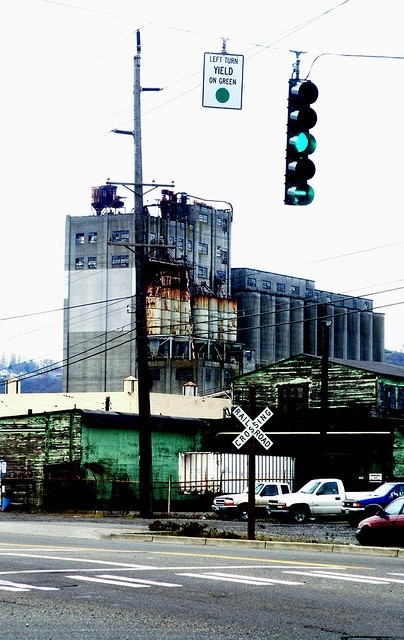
[212,482,292,520]
[267,478,368,522]
[342,482,404,526]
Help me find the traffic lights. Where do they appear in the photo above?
[283,79,317,206]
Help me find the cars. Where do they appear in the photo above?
[356,496,404,546]
